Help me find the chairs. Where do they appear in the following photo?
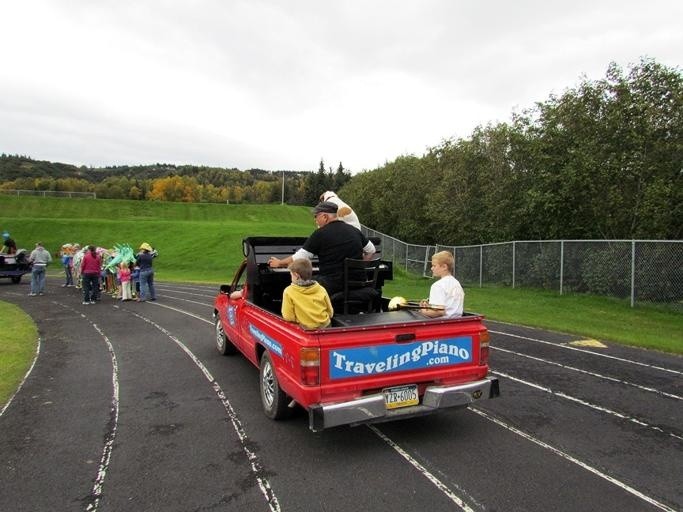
[332,256,382,317]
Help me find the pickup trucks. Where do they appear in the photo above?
[0,249,34,284]
[212,256,501,436]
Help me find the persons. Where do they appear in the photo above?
[267,201,376,295]
[419,250,465,322]
[25,241,52,296]
[0,231,17,255]
[58,240,159,306]
[280,257,335,331]
[228,288,243,300]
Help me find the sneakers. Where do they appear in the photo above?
[99,286,156,303]
[82,301,89,305]
[28,293,37,297]
[61,283,82,289]
[90,298,95,304]
[39,292,44,296]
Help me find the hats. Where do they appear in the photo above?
[311,201,338,214]
[62,244,81,250]
[139,243,153,252]
